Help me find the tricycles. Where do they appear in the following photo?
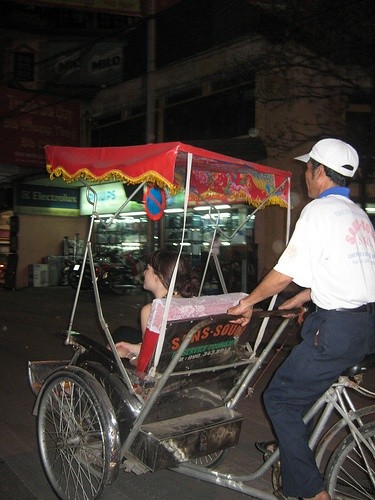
[27,142,375,500]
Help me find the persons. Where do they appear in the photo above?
[225,139,375,500]
[207,233,221,282]
[106,248,194,367]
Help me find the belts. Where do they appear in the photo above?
[316,302,375,312]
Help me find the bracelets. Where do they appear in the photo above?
[125,352,131,358]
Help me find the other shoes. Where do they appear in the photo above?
[254,440,281,460]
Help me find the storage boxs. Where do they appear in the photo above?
[28,263,49,287]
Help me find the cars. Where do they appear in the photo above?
[69,245,148,295]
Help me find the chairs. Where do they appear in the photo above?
[133,291,249,383]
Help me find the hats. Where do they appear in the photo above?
[293,138,359,178]
